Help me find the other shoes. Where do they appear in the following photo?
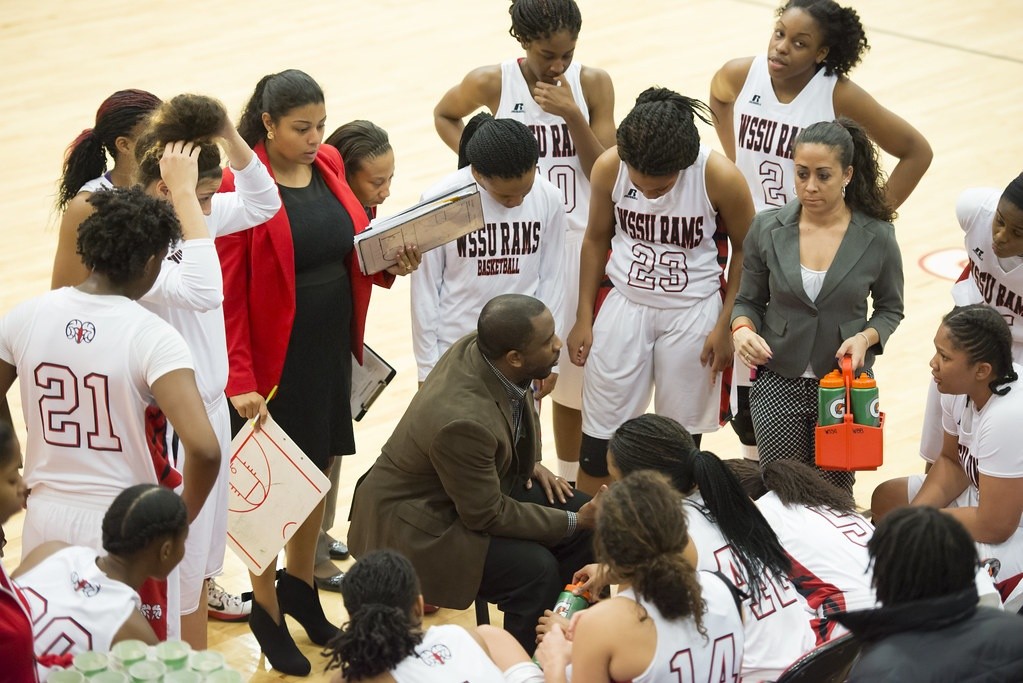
[316,570,347,592]
[328,539,350,559]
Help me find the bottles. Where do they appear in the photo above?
[818,368,881,431]
[530,581,591,670]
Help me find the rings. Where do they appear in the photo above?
[556,478,558,480]
[745,353,750,360]
[406,265,412,270]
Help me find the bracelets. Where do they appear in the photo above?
[731,324,752,335]
[857,332,870,349]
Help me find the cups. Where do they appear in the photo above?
[46,639,241,683]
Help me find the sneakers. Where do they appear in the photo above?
[204,575,252,621]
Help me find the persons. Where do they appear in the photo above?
[0,0,1023,683]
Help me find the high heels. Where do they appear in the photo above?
[242,590,311,676]
[274,567,347,650]
